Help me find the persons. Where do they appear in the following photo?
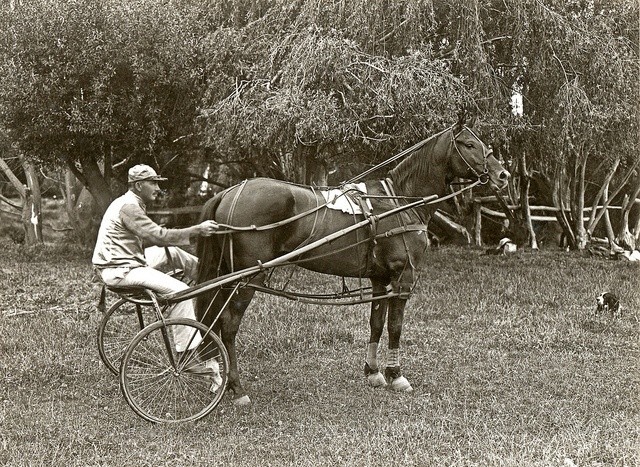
[93,164,220,376]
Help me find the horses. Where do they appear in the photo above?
[191,109,512,408]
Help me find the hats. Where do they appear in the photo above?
[128,164,168,182]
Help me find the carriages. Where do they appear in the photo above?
[97,115,511,424]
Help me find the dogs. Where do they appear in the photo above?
[594,290,627,317]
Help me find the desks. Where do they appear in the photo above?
[146,205,202,229]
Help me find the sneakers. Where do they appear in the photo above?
[178,350,214,374]
